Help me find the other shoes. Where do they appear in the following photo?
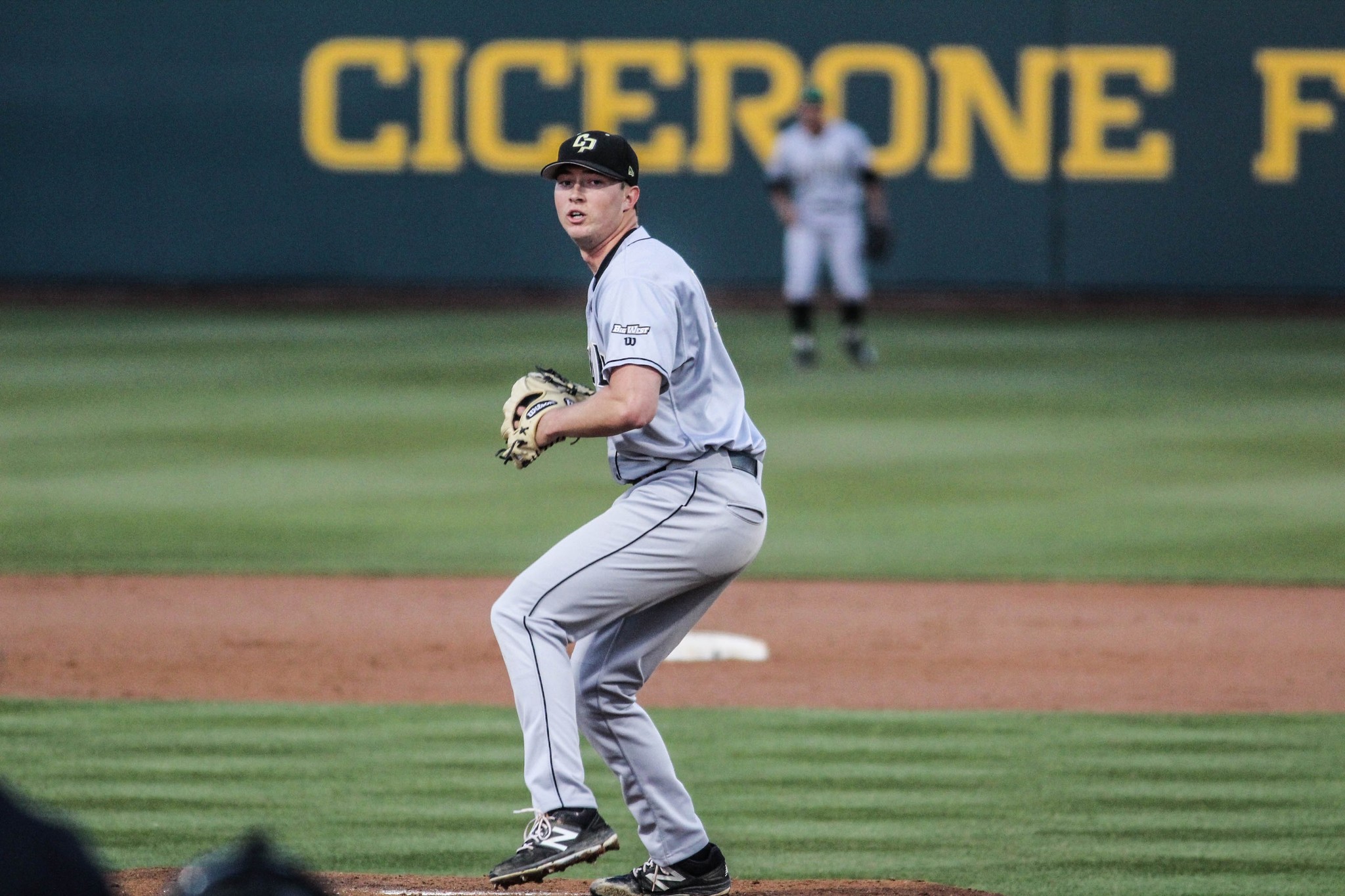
[786,331,815,372]
[842,331,872,368]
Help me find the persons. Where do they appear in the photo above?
[486,131,765,895]
[763,84,886,370]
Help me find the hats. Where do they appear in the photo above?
[542,130,640,184]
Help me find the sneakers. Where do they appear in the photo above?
[486,806,618,890]
[591,844,731,896]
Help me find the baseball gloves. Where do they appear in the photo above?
[500,370,578,472]
[866,224,890,265]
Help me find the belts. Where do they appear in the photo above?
[727,451,756,475]
